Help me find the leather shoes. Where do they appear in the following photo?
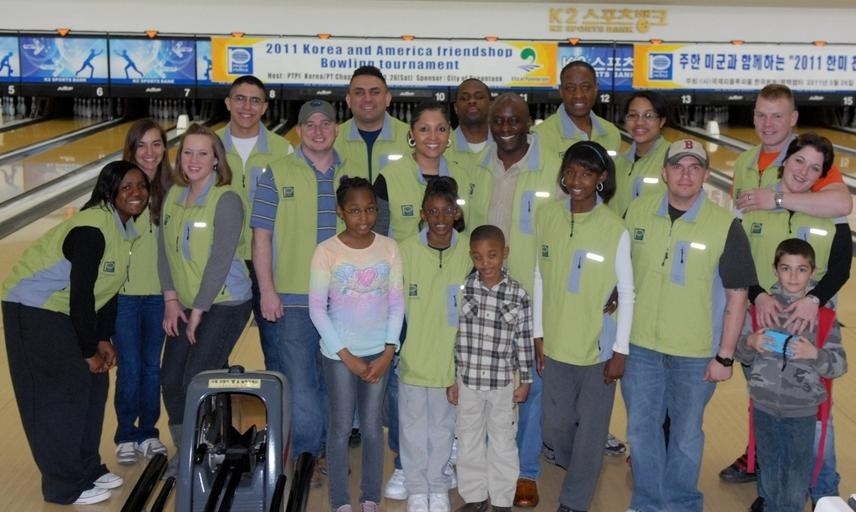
[513,478,539,507]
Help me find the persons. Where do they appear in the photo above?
[444,78,496,219]
[308,177,405,511]
[620,93,675,221]
[530,60,625,454]
[620,137,759,512]
[114,119,180,465]
[331,66,417,447]
[733,238,847,511]
[0,161,149,504]
[741,132,848,511]
[215,75,289,371]
[719,83,853,483]
[533,142,635,511]
[453,225,532,512]
[155,124,253,474]
[482,92,540,509]
[248,100,374,470]
[376,101,475,500]
[398,176,474,512]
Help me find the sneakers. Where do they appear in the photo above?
[72,486,112,505]
[319,458,351,476]
[719,447,758,483]
[429,493,450,512]
[115,442,138,464]
[383,468,408,501]
[359,500,377,512]
[406,494,429,512]
[444,462,457,491]
[93,471,123,489]
[491,506,511,512]
[605,433,626,455]
[336,504,352,512]
[139,436,168,459]
[309,464,324,489]
[455,501,488,512]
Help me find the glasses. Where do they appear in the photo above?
[428,207,456,217]
[624,111,658,121]
[231,95,264,106]
[343,206,375,216]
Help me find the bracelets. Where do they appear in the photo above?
[164,298,179,302]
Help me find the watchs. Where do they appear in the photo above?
[806,293,820,305]
[716,355,735,367]
[774,191,784,208]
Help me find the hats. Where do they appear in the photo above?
[298,99,336,123]
[663,139,709,169]
